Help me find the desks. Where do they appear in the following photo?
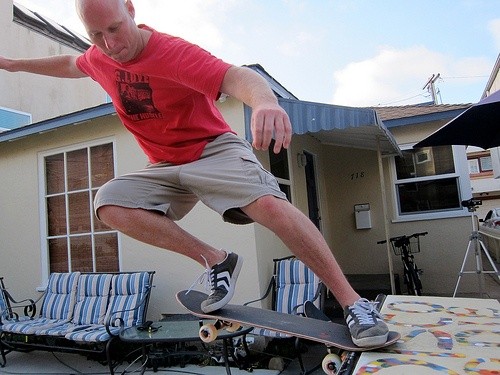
[336,293,500,375]
[119,320,255,375]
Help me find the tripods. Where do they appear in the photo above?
[453,207,500,298]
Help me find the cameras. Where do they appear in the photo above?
[462,199,482,207]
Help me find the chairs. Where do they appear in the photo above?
[245,256,334,375]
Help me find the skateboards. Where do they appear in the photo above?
[176,290,403,375]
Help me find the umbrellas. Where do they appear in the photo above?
[412,88,500,150]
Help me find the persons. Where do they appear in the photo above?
[0,0,389,346]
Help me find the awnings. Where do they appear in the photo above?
[244,97,403,158]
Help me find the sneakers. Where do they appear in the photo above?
[185,249,243,313]
[343,298,388,347]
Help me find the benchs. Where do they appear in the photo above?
[0,270,157,375]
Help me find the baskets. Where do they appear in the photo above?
[391,236,420,255]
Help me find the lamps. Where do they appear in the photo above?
[296,153,307,168]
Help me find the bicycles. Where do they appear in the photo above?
[377,232,428,296]
